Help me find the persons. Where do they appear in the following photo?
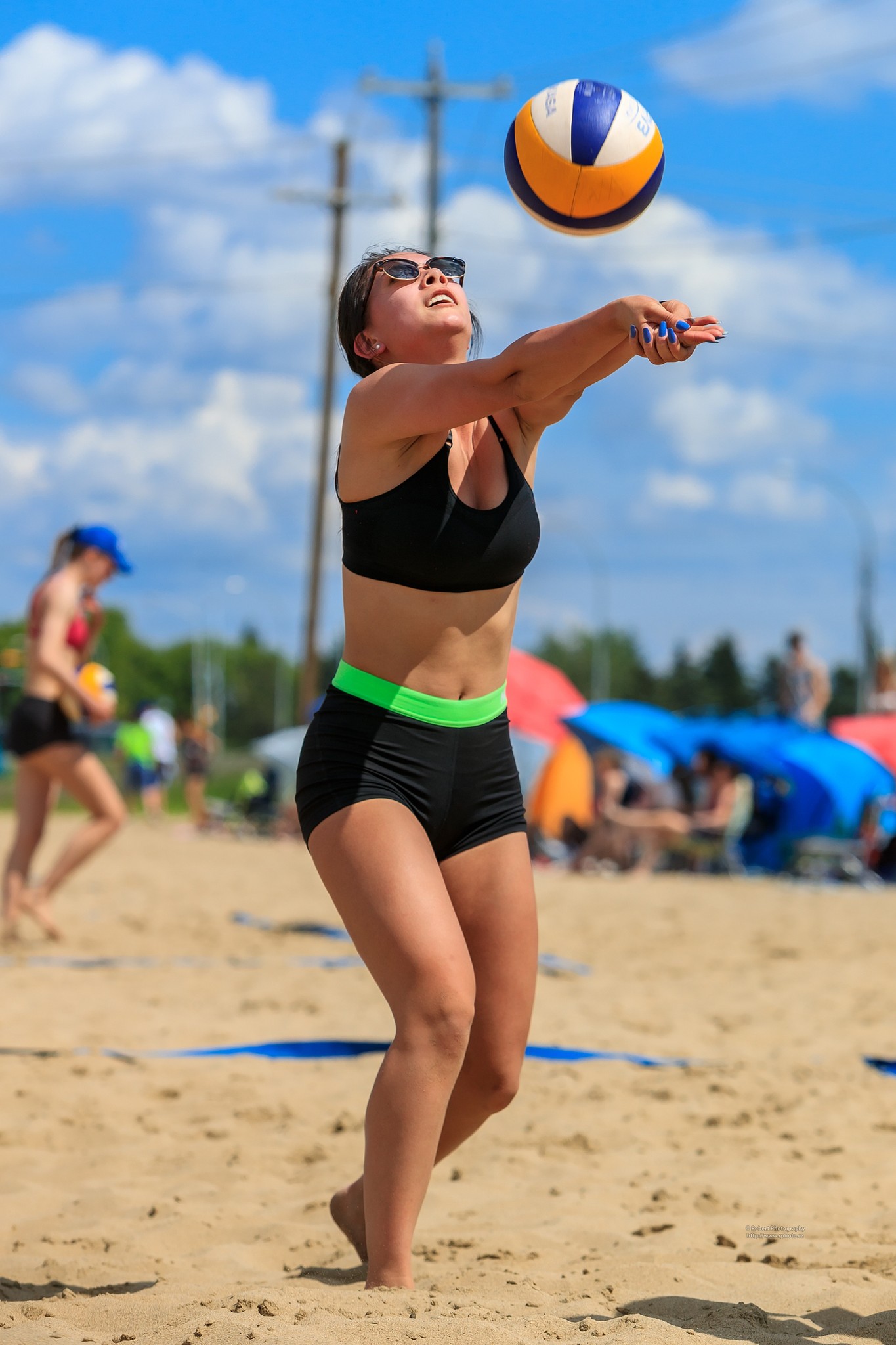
[0,527,275,949]
[500,619,830,879]
[294,250,729,1289]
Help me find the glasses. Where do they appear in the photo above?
[357,256,466,333]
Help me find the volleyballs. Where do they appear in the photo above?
[503,78,665,236]
[60,661,119,725]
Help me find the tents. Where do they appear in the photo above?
[500,644,896,867]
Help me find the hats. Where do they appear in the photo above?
[78,523,136,575]
